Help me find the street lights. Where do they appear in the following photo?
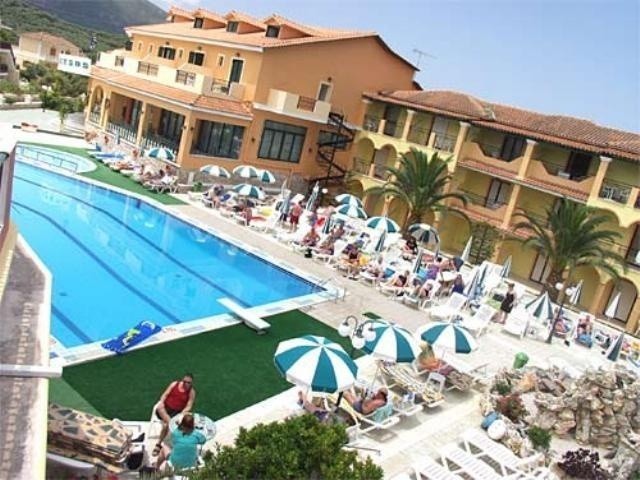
[314,187,328,205]
[546,279,576,343]
[335,315,376,413]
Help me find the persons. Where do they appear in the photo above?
[342,384,387,414]
[297,390,356,426]
[113,147,640,354]
[152,373,196,457]
[151,415,207,471]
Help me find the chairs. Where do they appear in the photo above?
[299,345,494,441]
[501,275,640,367]
[85,143,181,194]
[145,398,220,477]
[193,183,498,343]
[380,422,557,480]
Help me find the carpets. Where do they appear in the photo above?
[48,309,365,423]
[18,141,186,206]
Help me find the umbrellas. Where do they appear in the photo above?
[274,335,360,408]
[417,321,480,375]
[352,319,423,395]
